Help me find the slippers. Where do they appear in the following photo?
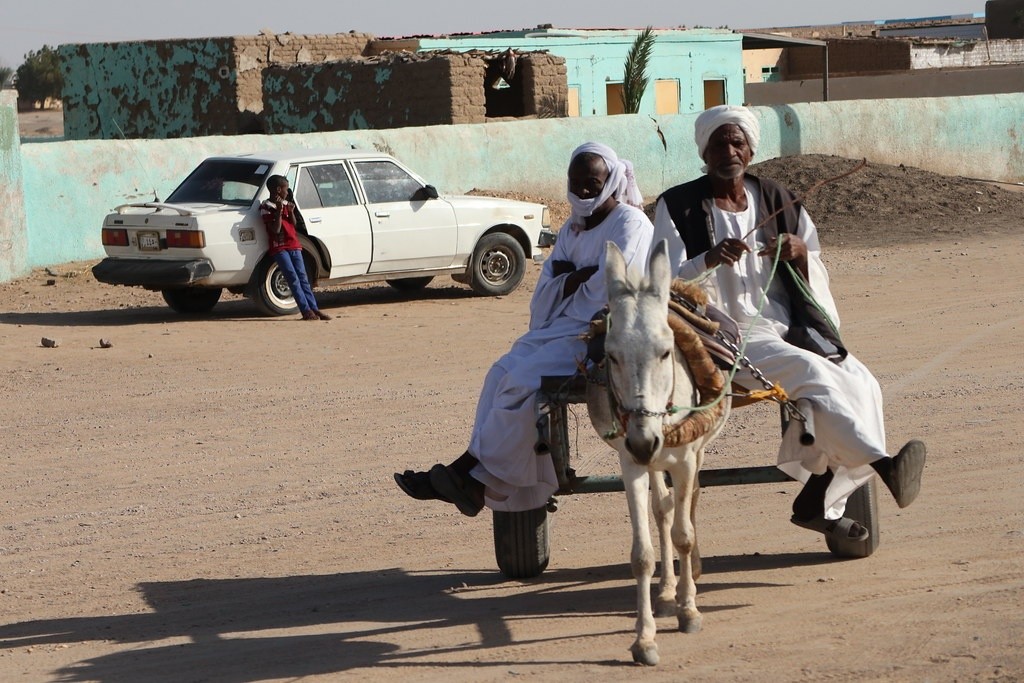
[395,463,484,517]
[790,511,869,542]
[888,439,926,508]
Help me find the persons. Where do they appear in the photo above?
[392,143,654,519]
[258,174,333,321]
[644,105,925,543]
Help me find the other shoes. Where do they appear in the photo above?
[303,310,333,320]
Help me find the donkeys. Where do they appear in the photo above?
[585,239,734,667]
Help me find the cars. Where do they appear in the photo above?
[89,151,555,318]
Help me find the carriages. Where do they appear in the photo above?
[491,237,877,664]
